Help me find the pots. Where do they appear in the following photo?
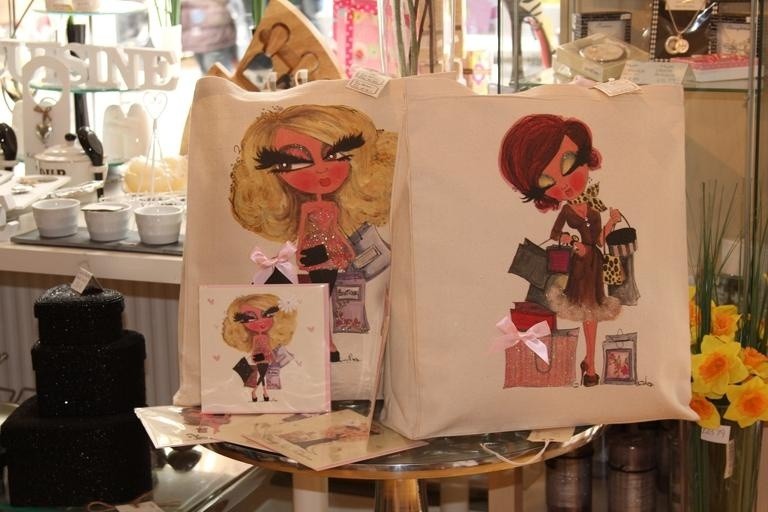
[31,136,110,198]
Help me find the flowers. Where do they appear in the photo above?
[685,181,766,432]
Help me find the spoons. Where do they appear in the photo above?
[78,126,103,201]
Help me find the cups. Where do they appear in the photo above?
[85,203,130,241]
[32,198,80,237]
[132,205,182,245]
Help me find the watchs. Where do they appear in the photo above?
[570,235,579,246]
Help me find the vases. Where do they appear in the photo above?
[687,404,761,510]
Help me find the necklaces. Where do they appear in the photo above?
[665,0,705,55]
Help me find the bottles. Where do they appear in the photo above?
[549,451,590,512]
[609,435,654,512]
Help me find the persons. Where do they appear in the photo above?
[229,104,398,362]
[500,114,622,387]
[223,293,297,402]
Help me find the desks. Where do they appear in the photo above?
[202,401,598,512]
